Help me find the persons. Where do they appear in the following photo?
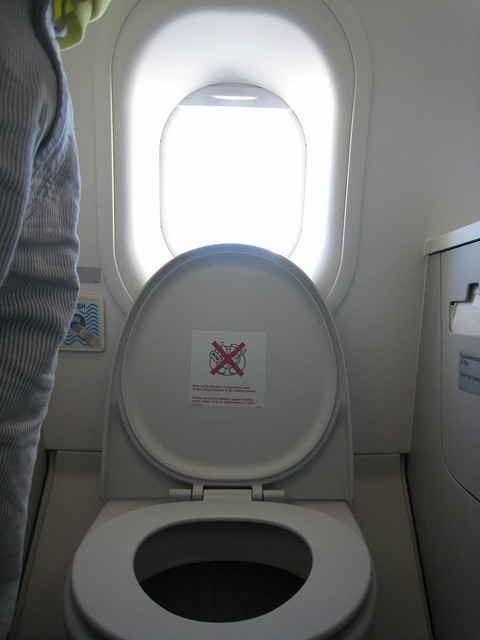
[1,1,80,639]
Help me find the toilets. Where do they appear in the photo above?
[63,243,378,640]
